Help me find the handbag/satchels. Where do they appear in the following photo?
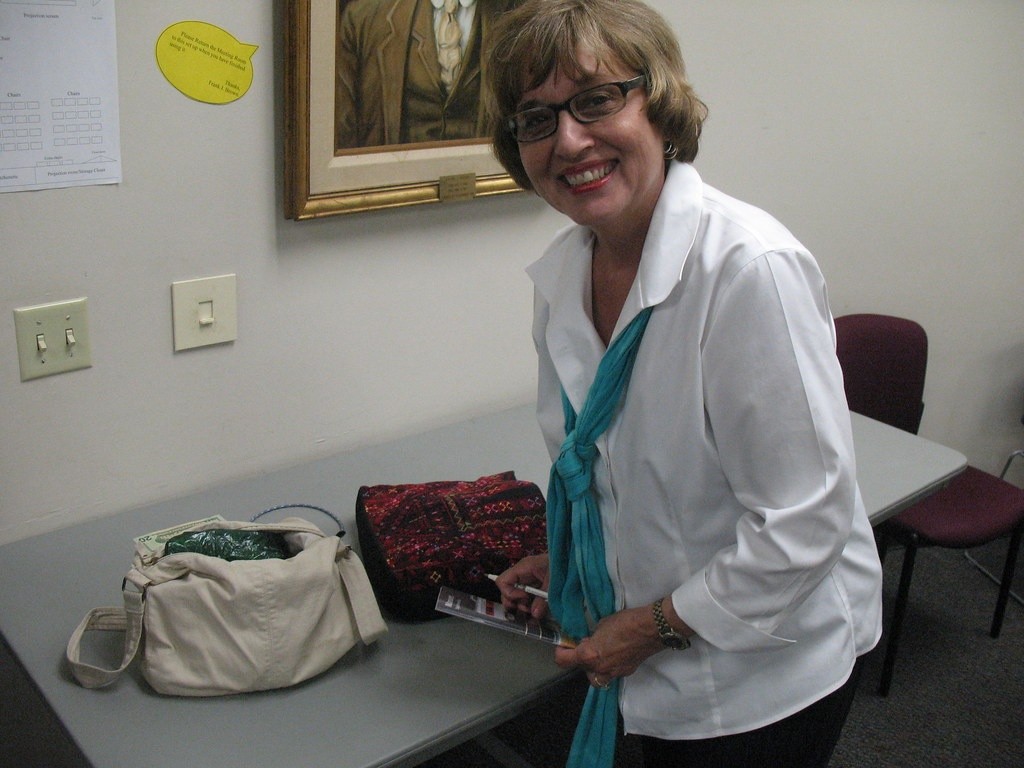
[355,470,549,624]
[65,519,387,697]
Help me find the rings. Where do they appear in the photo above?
[594,676,608,688]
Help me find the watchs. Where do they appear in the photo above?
[653,597,690,650]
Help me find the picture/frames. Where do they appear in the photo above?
[286,0,530,222]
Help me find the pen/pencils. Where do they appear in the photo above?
[482,572,549,599]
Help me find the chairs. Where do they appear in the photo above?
[833,312,1024,698]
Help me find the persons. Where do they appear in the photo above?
[485,0,884,768]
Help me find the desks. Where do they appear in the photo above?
[0,400,971,768]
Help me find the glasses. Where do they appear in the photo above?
[504,74,649,143]
